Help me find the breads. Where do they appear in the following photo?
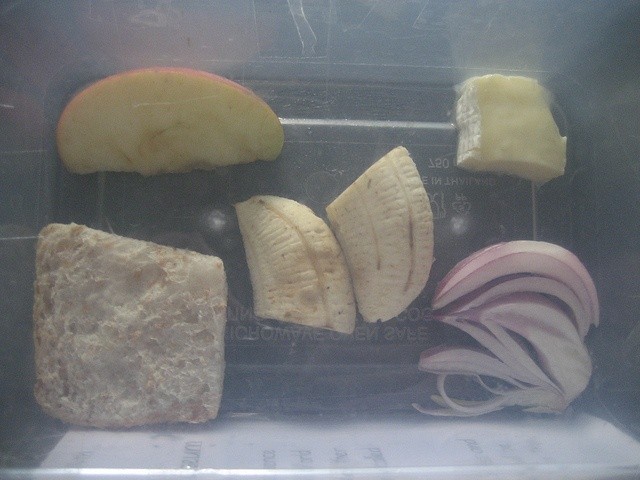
[27,219,231,436]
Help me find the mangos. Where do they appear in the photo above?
[48,64,286,181]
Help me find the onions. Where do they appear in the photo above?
[410,237,600,421]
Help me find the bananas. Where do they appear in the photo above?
[228,191,359,336]
[323,142,436,329]
